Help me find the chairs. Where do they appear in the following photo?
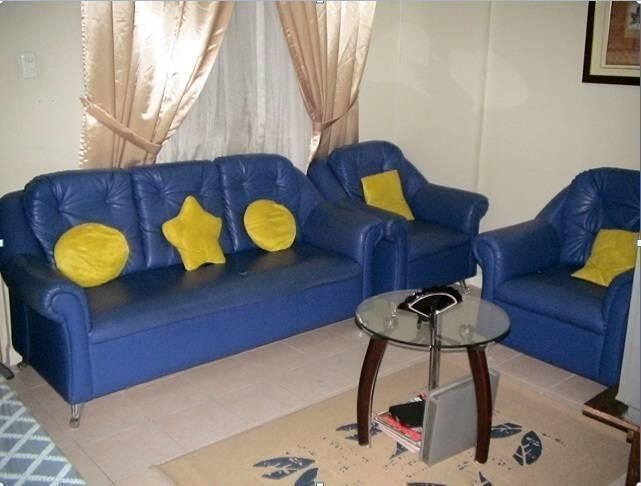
[471,167,641,388]
[306,140,489,293]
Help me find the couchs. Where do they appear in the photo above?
[0,153,384,428]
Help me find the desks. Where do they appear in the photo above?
[582,385,641,486]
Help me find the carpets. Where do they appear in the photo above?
[154,350,631,486]
[0,374,87,486]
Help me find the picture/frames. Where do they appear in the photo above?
[582,0,641,86]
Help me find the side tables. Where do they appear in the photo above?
[355,289,512,464]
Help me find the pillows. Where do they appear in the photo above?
[243,199,296,252]
[53,222,130,289]
[359,169,415,222]
[568,227,641,287]
[161,195,226,272]
